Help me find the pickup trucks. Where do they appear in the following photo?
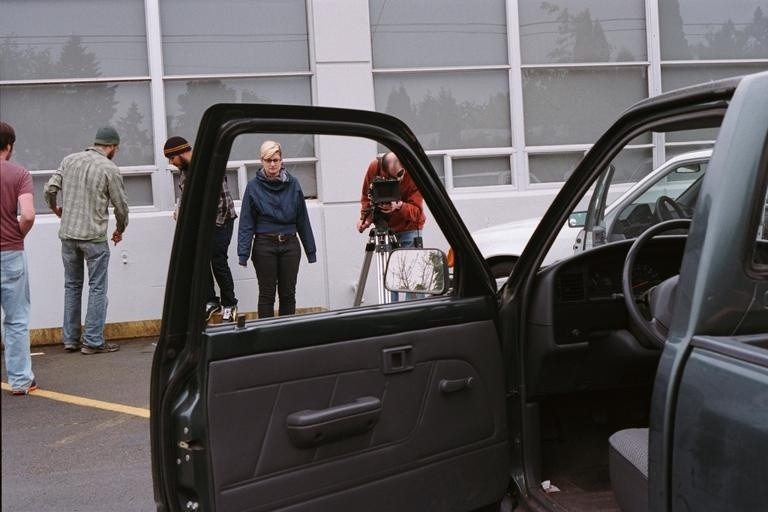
[148,64,768,511]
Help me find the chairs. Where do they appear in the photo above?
[606,426,650,511]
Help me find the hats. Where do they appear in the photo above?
[94,125,121,145]
[164,136,191,157]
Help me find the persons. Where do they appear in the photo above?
[42,126,129,354]
[357,153,426,301]
[237,141,317,318]
[164,136,239,324]
[1,121,38,395]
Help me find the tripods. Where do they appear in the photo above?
[353,209,417,308]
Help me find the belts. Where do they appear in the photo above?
[255,232,295,242]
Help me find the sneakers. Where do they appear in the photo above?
[204,297,221,321]
[64,339,83,351]
[79,340,120,355]
[220,301,237,322]
[13,377,38,395]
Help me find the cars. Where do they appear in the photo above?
[447,149,715,301]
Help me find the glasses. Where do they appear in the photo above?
[263,158,279,162]
